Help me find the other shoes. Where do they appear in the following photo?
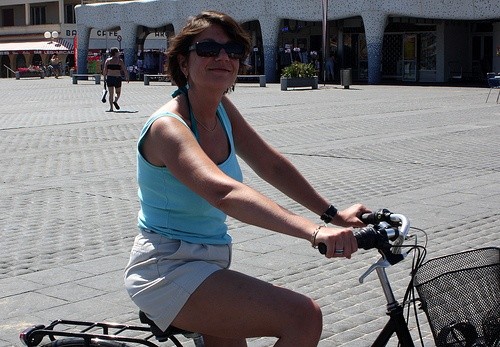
[105,110,113,112]
[114,101,120,110]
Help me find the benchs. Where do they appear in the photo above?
[16,71,45,78]
[144,74,176,86]
[71,73,100,84]
[236,75,266,86]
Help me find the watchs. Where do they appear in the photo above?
[320,205,337,223]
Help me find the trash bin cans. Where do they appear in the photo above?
[70,69,77,77]
[340,68,352,89]
[128,65,137,81]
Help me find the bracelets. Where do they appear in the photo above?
[311,223,328,250]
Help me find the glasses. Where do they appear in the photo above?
[188,38,246,59]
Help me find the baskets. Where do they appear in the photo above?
[413,247,500,347]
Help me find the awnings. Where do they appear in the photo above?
[0,38,74,55]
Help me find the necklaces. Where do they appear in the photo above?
[193,112,218,130]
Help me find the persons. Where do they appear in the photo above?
[326,47,336,82]
[51,53,64,79]
[103,48,130,112]
[123,11,372,347]
[136,56,144,81]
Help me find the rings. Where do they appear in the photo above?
[335,250,343,254]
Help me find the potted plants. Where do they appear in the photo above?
[280,62,318,90]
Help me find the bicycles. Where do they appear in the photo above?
[19,208,500,347]
